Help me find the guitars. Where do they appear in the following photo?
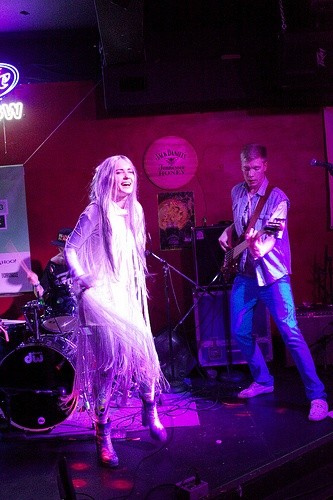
[218,222,283,284]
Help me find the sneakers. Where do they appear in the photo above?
[237,382,273,399]
[307,399,329,421]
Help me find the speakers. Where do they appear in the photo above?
[287,314,333,366]
[195,226,243,286]
[191,291,275,365]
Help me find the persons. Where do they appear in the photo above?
[0,318,9,352]
[218,143,328,421]
[27,228,76,316]
[64,155,170,467]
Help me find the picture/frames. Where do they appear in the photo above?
[156,192,197,252]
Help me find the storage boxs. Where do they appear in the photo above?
[192,285,274,377]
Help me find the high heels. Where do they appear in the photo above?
[141,400,167,442]
[96,416,120,467]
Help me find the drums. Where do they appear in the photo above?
[1,320,28,342]
[0,334,89,432]
[20,299,49,320]
[41,315,81,347]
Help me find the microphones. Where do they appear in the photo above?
[59,386,66,395]
[311,160,333,169]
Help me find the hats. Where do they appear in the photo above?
[50,228,74,247]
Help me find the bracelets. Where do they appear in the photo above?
[32,281,40,287]
